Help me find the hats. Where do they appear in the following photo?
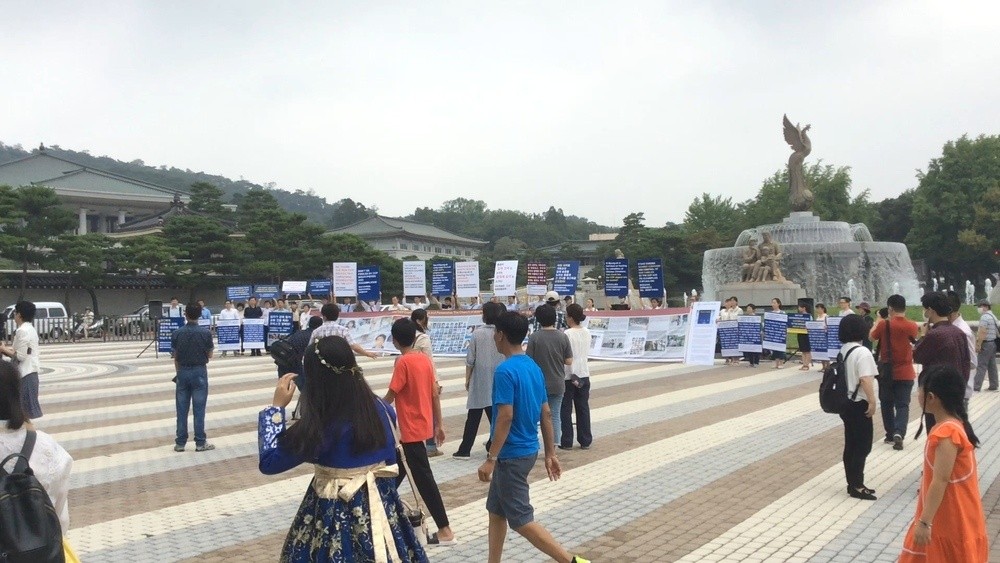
[855,301,872,313]
[546,291,559,301]
[973,299,992,306]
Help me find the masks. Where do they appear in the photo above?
[923,309,931,323]
[976,308,985,314]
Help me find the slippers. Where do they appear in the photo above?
[427,532,457,545]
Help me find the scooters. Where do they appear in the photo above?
[71,313,104,340]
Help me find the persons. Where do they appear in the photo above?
[164,280,517,356]
[454,291,594,563]
[715,297,874,372]
[1,299,73,563]
[372,333,386,349]
[563,288,672,310]
[345,320,357,331]
[171,301,216,451]
[741,231,781,283]
[614,249,624,259]
[82,307,94,339]
[837,292,1000,563]
[258,304,453,563]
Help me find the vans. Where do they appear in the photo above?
[2,302,67,339]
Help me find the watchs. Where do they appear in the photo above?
[487,452,497,460]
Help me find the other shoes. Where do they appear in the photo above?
[451,452,472,460]
[571,555,591,563]
[219,352,261,359]
[847,485,877,500]
[426,449,444,459]
[772,364,825,373]
[723,361,759,368]
[985,387,999,392]
[974,388,980,392]
[581,441,592,448]
[174,444,185,452]
[893,433,904,450]
[195,443,215,451]
[884,438,895,445]
[557,445,573,450]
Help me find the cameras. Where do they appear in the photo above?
[875,308,888,318]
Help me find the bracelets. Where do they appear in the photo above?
[919,519,932,529]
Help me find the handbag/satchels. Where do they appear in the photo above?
[270,338,302,372]
[874,361,892,386]
[994,337,1000,352]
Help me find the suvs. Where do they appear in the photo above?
[288,300,323,317]
[114,303,186,337]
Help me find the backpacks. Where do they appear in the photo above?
[819,346,861,414]
[0,426,65,563]
